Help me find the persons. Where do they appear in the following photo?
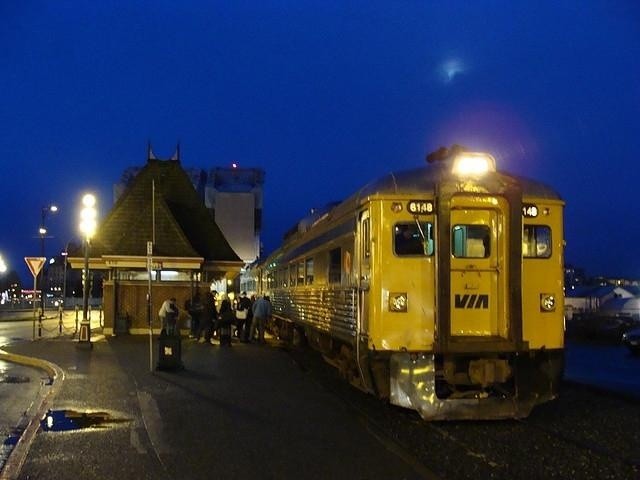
[155,288,276,347]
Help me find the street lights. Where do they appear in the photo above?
[39,201,59,318]
[76,191,97,345]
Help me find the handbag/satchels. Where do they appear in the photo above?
[236,309,247,319]
[214,314,226,330]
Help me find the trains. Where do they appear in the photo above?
[237,144,568,426]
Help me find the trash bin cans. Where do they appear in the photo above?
[118,315,128,335]
[158,336,182,371]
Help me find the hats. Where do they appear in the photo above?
[264,295,270,303]
[212,291,218,294]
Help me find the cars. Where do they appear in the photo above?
[622,325,640,354]
[568,317,629,339]
